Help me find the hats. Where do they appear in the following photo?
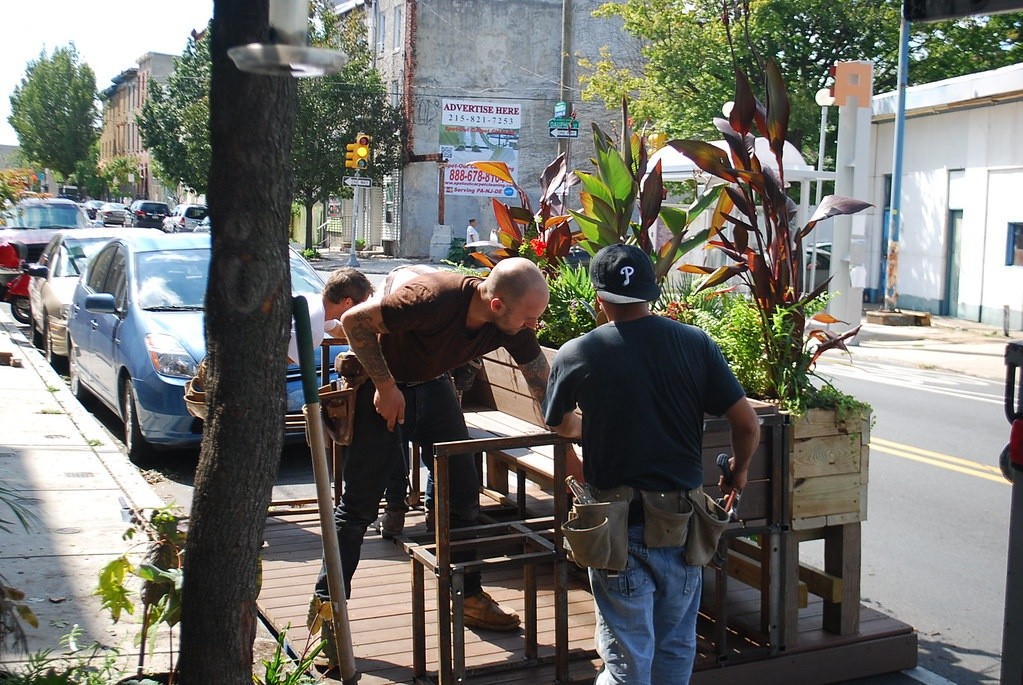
[590,243,660,304]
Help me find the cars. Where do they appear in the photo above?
[26,227,164,369]
[123,200,173,233]
[190,214,211,234]
[79,199,104,220]
[0,196,106,274]
[66,233,351,465]
[92,203,129,228]
[162,203,206,231]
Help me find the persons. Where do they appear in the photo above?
[197,267,376,390]
[315,256,551,665]
[467,219,479,252]
[369,264,441,536]
[542,243,761,685]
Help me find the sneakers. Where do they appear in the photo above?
[375,505,405,539]
[424,508,435,530]
[321,619,338,658]
[450,589,520,631]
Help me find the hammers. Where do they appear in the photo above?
[716,453,744,521]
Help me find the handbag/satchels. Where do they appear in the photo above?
[685,486,729,567]
[640,490,694,548]
[561,486,634,570]
[301,374,369,446]
[183,358,208,419]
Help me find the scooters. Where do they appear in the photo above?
[0,239,34,323]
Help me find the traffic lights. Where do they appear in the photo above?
[344,144,357,168]
[353,134,370,170]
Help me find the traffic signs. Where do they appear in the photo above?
[342,176,372,188]
[548,127,581,138]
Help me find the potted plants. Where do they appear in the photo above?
[458,25,872,530]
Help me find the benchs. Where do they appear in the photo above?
[392,337,797,685]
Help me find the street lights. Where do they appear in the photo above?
[808,88,835,298]
